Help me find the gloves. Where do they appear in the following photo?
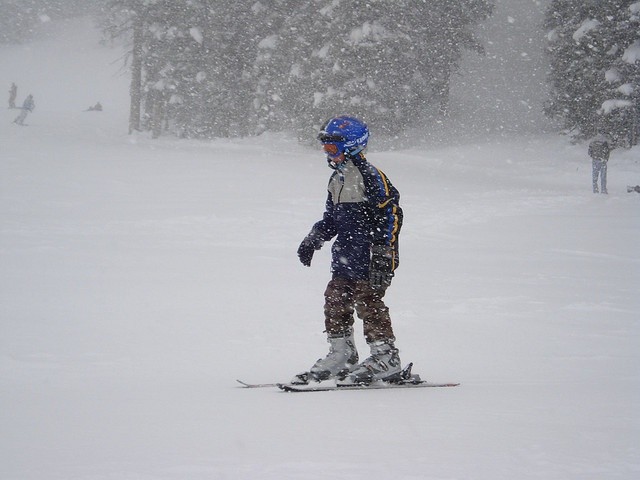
[368,245,395,290]
[297,229,331,267]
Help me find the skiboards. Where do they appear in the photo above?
[235,363,459,392]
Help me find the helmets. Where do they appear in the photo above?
[317,116,370,170]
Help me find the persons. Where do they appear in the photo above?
[588,132,610,194]
[9,83,17,106]
[14,94,35,124]
[292,115,403,382]
